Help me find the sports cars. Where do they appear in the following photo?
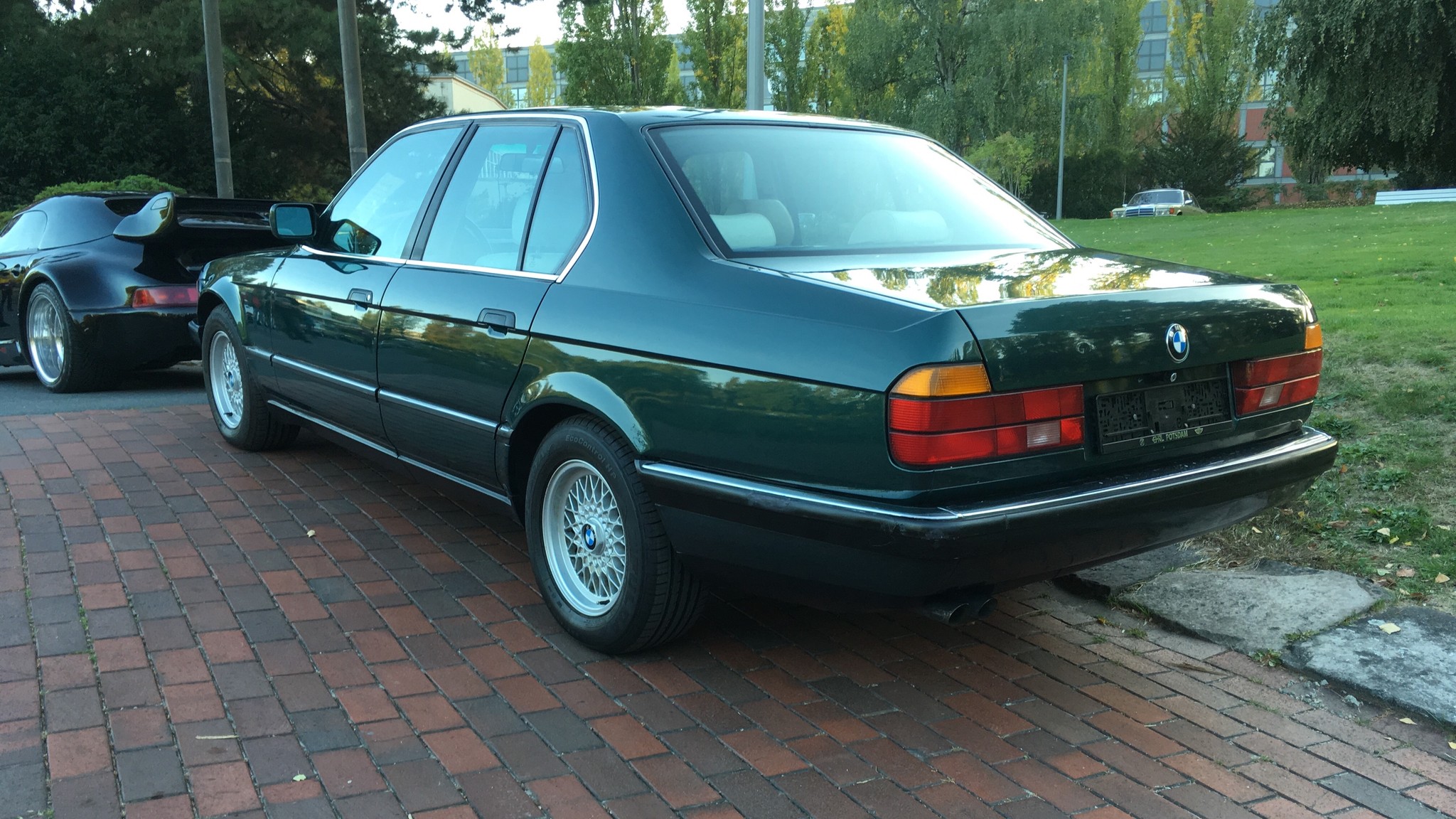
[0,189,330,395]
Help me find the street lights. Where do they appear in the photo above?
[1056,53,1074,219]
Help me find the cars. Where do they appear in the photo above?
[192,105,1340,664]
[1110,188,1209,219]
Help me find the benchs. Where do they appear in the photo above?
[1373,189,1455,204]
